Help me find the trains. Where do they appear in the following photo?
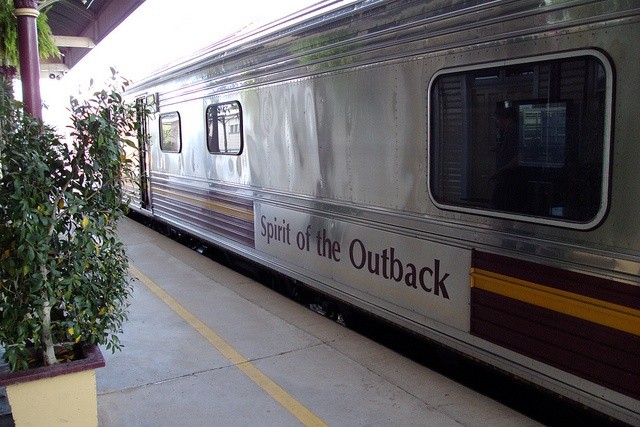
[74,2,638,427]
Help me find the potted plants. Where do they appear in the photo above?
[0,66,156,427]
